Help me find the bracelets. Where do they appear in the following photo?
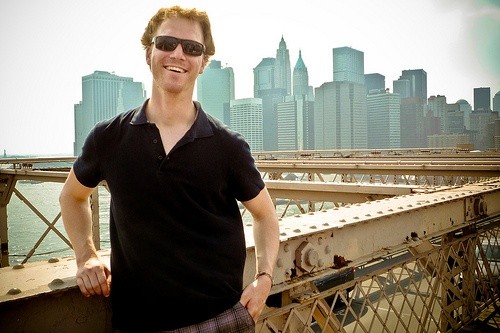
[255,271,274,290]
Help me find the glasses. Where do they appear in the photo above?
[149,34,207,57]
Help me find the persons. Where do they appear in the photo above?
[58,6,281,333]
